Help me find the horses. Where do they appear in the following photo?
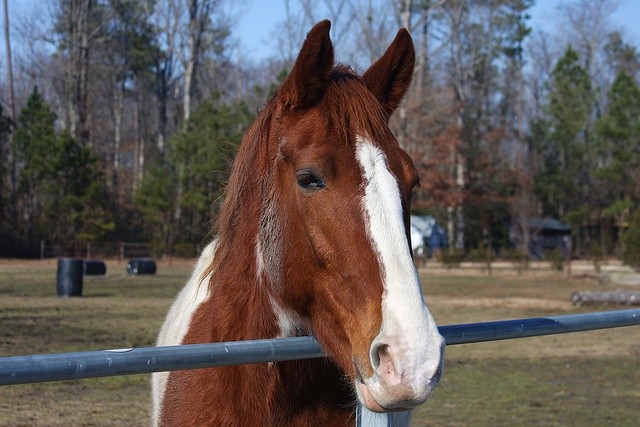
[152,19,445,427]
